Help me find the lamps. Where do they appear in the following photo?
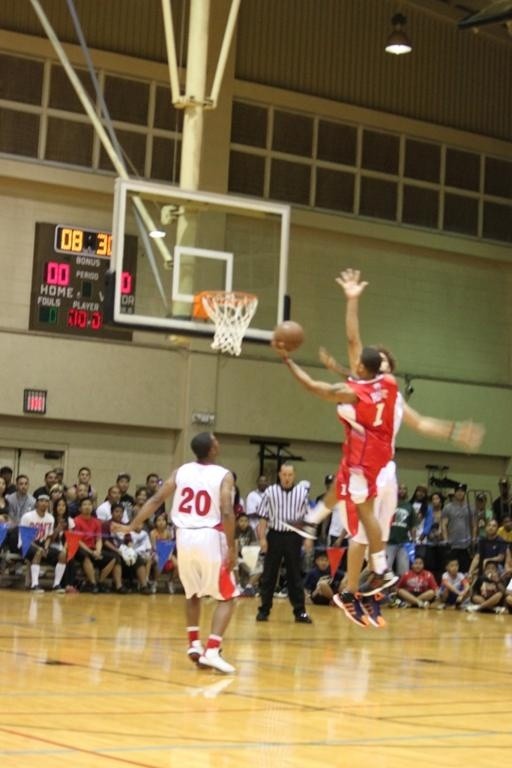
[385,0,413,56]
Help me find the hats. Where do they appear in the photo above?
[117,472,130,482]
[477,494,486,500]
[299,480,310,488]
[53,468,63,474]
[455,483,466,489]
[325,474,334,484]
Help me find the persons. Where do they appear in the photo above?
[298,473,510,615]
[256,462,312,623]
[332,267,484,629]
[275,340,405,597]
[229,472,289,597]
[270,340,405,597]
[2,465,185,595]
[111,432,243,673]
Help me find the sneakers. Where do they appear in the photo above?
[333,592,373,628]
[167,582,174,593]
[256,613,266,621]
[399,600,412,607]
[31,585,45,593]
[359,595,387,627]
[295,613,312,624]
[141,587,152,594]
[91,584,99,592]
[355,568,398,596]
[199,648,236,673]
[117,585,128,593]
[282,514,319,540]
[496,606,506,614]
[67,585,76,593]
[419,600,429,609]
[52,585,66,593]
[187,640,213,669]
[99,583,110,592]
[466,605,480,612]
[151,582,158,593]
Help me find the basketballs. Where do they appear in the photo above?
[274,321,304,351]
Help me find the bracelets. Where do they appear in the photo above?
[448,419,456,440]
